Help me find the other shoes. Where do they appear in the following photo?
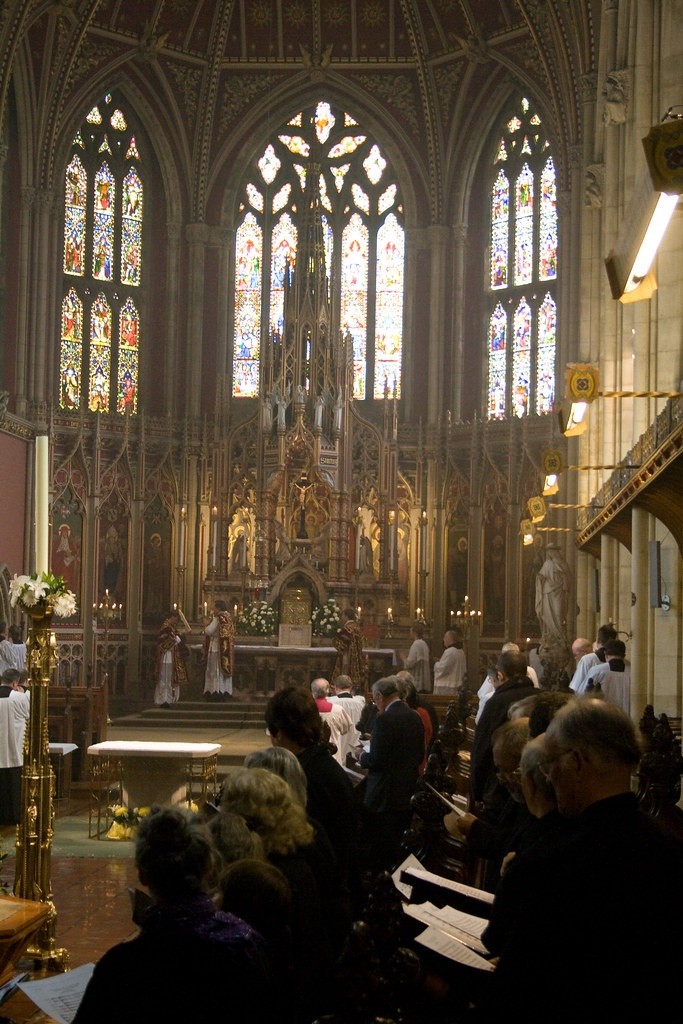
[162,701,169,708]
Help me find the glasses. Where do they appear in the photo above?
[372,693,379,705]
[496,771,515,783]
[514,767,528,785]
[538,749,574,777]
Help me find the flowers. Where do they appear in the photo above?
[311,598,342,636]
[106,805,151,827]
[7,571,80,620]
[239,600,276,635]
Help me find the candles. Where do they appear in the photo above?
[423,510,426,519]
[92,589,122,620]
[357,506,361,517]
[213,506,217,516]
[234,604,238,617]
[450,595,482,627]
[417,608,421,619]
[204,601,208,616]
[388,608,391,620]
[180,507,184,515]
[390,511,394,520]
[358,606,361,619]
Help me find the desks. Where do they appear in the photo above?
[86,741,223,840]
[49,743,79,806]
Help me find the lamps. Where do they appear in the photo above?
[604,120,683,306]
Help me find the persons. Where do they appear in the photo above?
[74,651,683,1024]
[433,629,466,695]
[475,624,634,716]
[534,544,574,655]
[329,609,368,696]
[203,600,235,702]
[401,624,431,694]
[0,622,32,827]
[152,612,189,708]
